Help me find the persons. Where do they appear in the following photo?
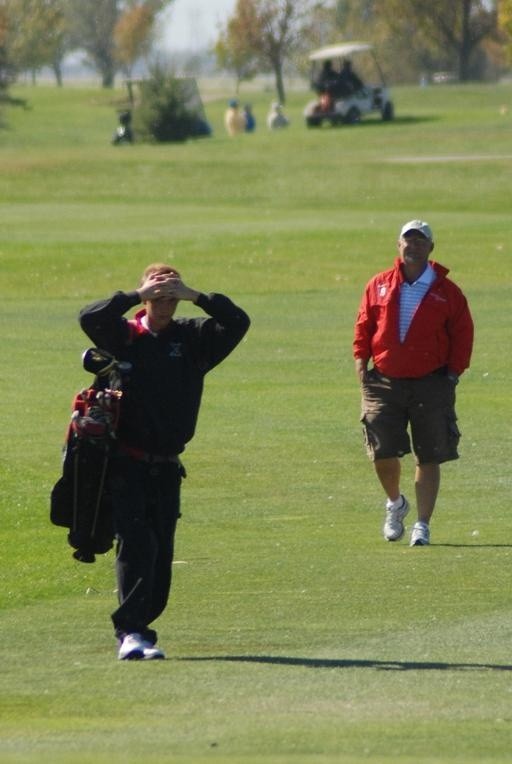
[341,58,365,91]
[225,97,247,135]
[267,101,288,129]
[50,260,251,661]
[351,218,474,551]
[316,57,340,84]
[241,102,256,132]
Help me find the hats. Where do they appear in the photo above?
[402,220,431,238]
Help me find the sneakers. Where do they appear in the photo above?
[409,521,429,546]
[384,493,409,541]
[119,632,163,660]
